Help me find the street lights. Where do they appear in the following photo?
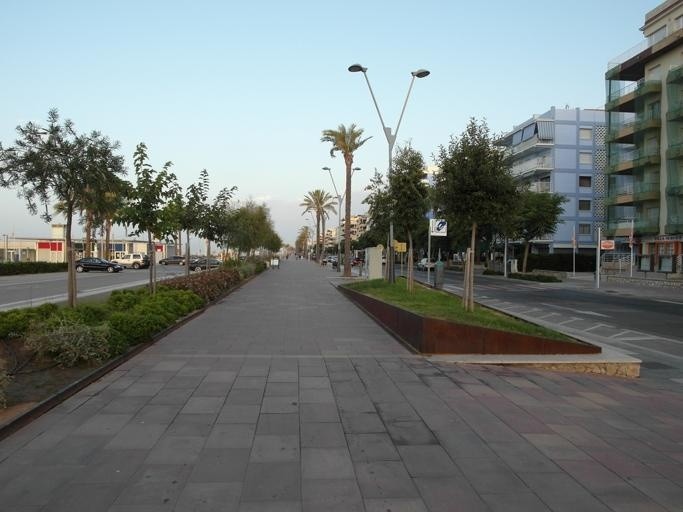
[321,165,361,269]
[346,63,429,283]
[300,208,330,261]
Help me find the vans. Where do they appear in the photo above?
[114,251,151,270]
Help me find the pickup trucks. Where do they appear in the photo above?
[413,256,438,272]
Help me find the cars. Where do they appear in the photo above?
[159,253,186,267]
[68,254,123,274]
[188,257,227,273]
